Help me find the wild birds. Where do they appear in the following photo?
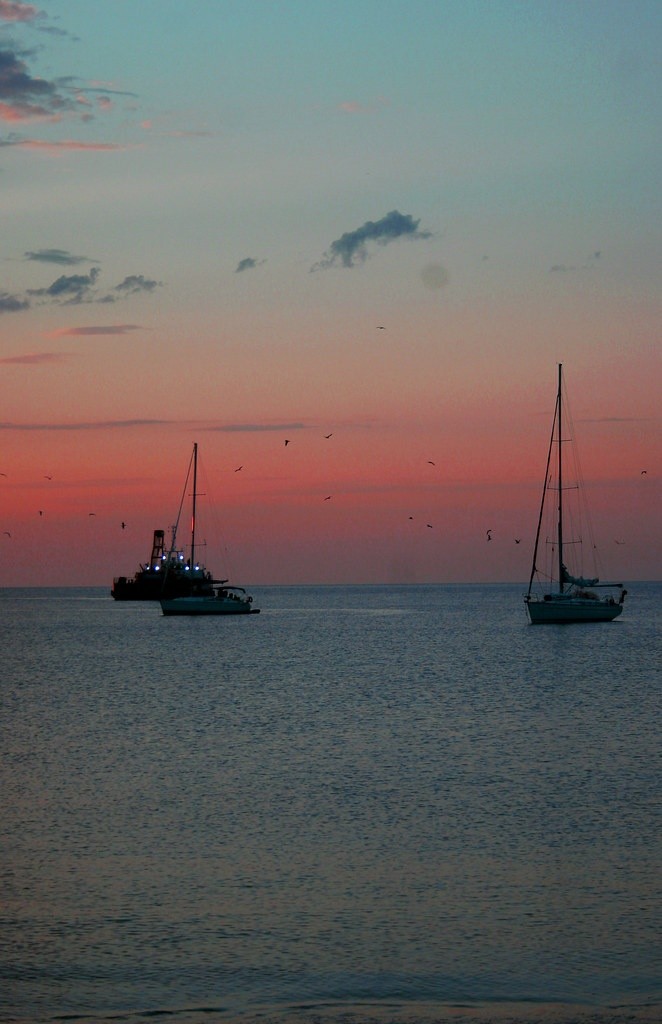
[89,513,95,516]
[38,510,43,516]
[377,326,385,330]
[43,474,56,480]
[614,540,626,544]
[641,471,647,474]
[324,433,332,438]
[0,473,7,477]
[408,517,432,528]
[428,461,435,467]
[235,465,243,472]
[322,495,332,502]
[285,440,290,446]
[515,539,520,544]
[4,532,11,537]
[121,522,126,529]
[486,529,492,541]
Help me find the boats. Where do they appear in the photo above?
[160,585,261,616]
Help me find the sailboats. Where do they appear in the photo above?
[110,442,237,600]
[521,363,628,623]
